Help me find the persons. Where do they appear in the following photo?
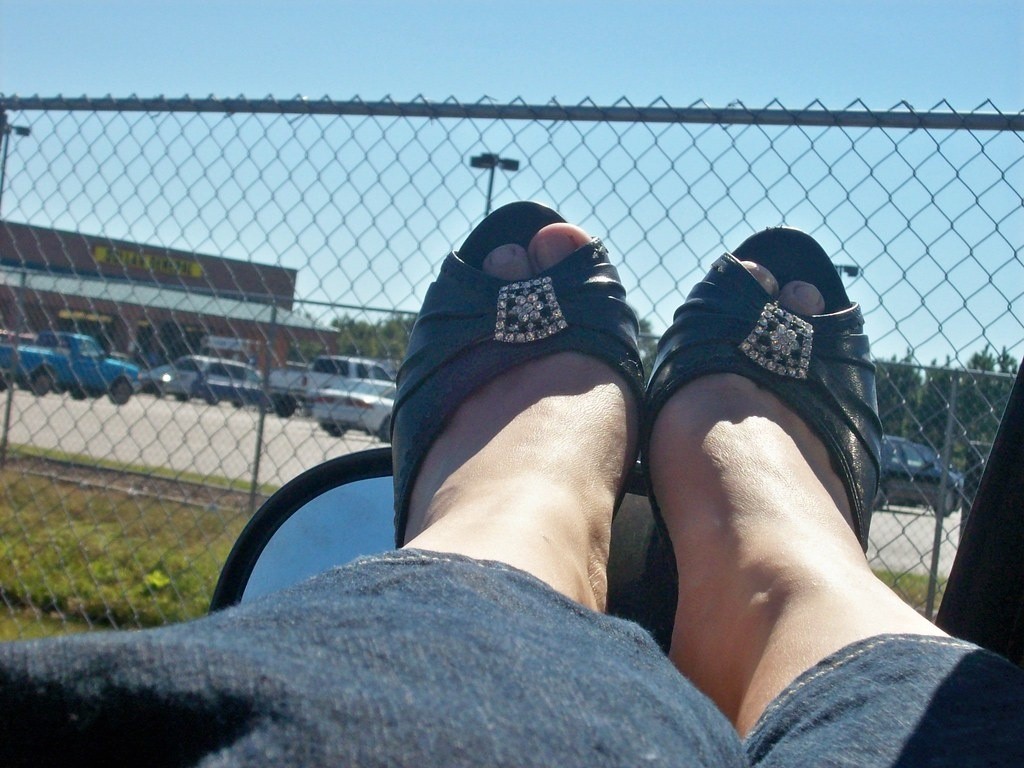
[0,201,1024,768]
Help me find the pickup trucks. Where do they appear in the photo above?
[0,332,144,406]
[268,355,397,418]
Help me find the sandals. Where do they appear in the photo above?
[641,229,883,656]
[390,201,646,552]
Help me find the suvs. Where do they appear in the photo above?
[872,436,962,517]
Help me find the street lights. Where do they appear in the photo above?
[470,152,522,221]
[1,123,34,204]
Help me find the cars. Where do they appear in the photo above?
[314,380,399,442]
[143,356,263,407]
[940,437,992,502]
